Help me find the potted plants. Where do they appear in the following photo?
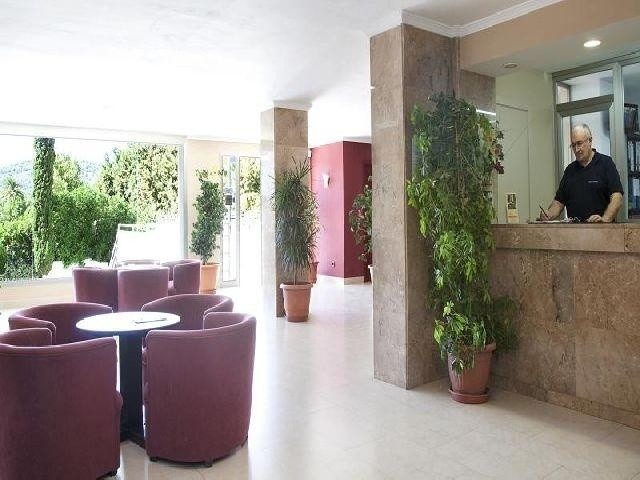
[189,166,228,295]
[401,90,518,405]
[348,173,373,286]
[268,156,318,322]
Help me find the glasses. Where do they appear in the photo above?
[568,140,588,151]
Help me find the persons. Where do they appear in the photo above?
[538,122,624,223]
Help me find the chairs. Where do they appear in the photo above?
[75,258,200,309]
[141,294,233,329]
[9,303,113,343]
[143,312,257,466]
[2,328,125,478]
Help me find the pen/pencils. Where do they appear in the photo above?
[539,206,550,219]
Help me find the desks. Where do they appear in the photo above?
[76,311,180,448]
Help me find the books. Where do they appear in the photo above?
[527,220,570,224]
[625,106,640,219]
